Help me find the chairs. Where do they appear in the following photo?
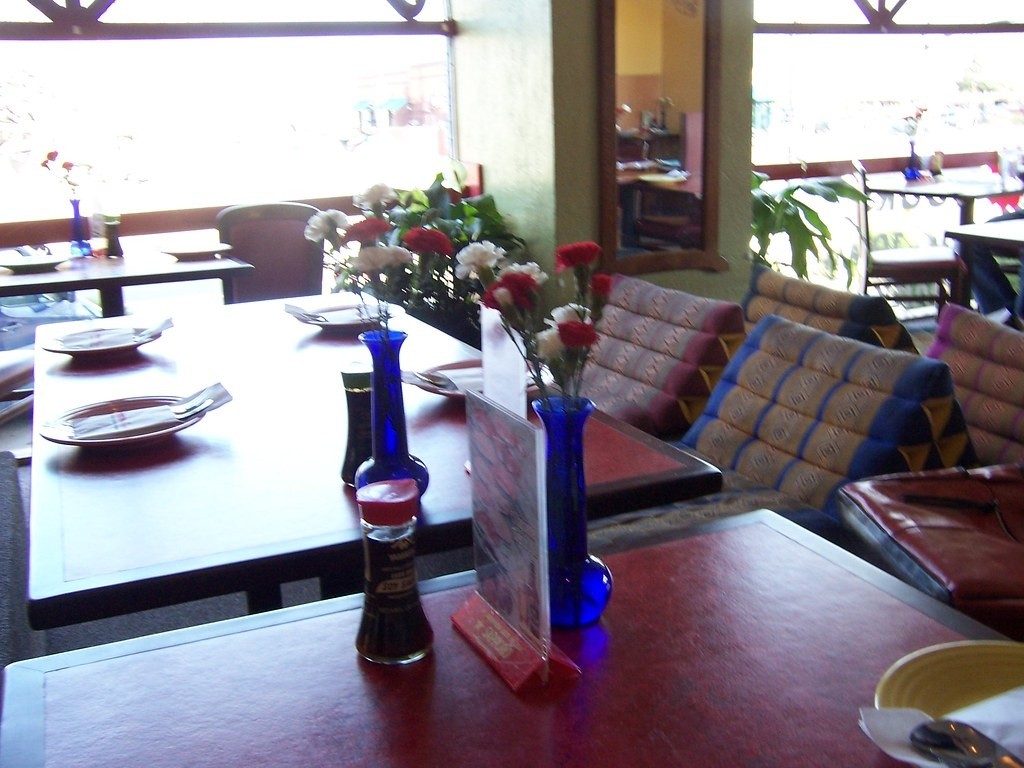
[214,202,326,302]
[583,157,1024,633]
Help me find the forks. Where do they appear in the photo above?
[171,389,211,414]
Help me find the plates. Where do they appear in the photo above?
[873,638,1024,725]
[39,396,206,452]
[161,242,233,260]
[41,326,163,361]
[303,301,406,336]
[419,358,556,399]
[0,255,69,273]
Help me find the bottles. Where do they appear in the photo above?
[103,214,124,259]
[341,366,375,489]
[354,477,435,666]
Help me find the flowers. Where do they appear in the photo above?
[356,144,522,310]
[454,241,617,415]
[41,148,94,199]
[306,188,453,337]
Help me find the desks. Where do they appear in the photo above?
[27,318,719,633]
[0,243,254,322]
[0,510,1024,768]
[866,167,1022,263]
[945,221,1024,331]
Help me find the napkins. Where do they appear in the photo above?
[857,683,1024,768]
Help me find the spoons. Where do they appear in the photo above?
[910,720,1024,768]
[414,371,458,390]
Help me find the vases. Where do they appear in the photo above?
[401,299,483,348]
[70,200,93,255]
[531,394,613,628]
[354,331,430,501]
